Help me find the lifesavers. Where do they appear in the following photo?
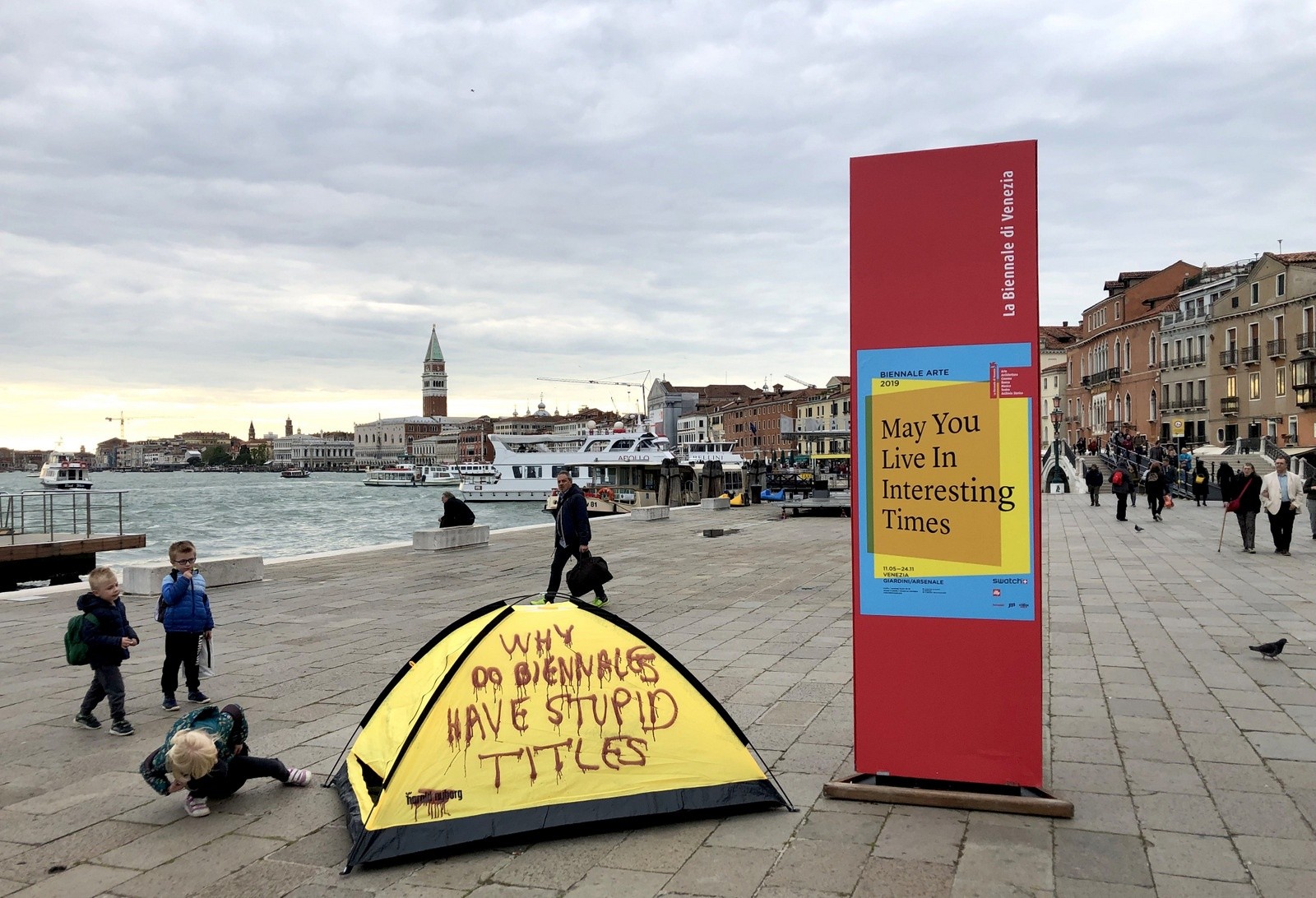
[61,461,69,468]
[596,489,614,501]
[80,462,87,469]
[417,474,421,478]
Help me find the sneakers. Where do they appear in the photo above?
[73,711,103,729]
[283,768,312,785]
[110,720,135,735]
[162,699,180,710]
[188,691,211,703]
[184,796,211,816]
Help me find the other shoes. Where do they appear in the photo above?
[531,597,546,605]
[1245,547,1257,552]
[1091,502,1100,507]
[1154,513,1163,521]
[1275,548,1290,556]
[1197,505,1201,506]
[592,595,609,608]
[1203,503,1207,506]
[1119,519,1128,521]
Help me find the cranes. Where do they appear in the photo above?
[536,369,651,418]
[105,410,172,439]
[784,373,817,389]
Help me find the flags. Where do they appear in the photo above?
[750,422,756,435]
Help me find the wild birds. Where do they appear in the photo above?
[60,436,63,440]
[55,442,64,446]
[471,89,474,92]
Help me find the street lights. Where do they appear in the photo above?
[1049,394,1064,484]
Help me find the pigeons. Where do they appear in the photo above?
[1249,638,1290,661]
[1089,452,1091,456]
[1238,460,1240,463]
[1135,525,1145,532]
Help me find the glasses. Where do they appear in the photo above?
[175,558,197,565]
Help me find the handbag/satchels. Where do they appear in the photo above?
[1194,467,1205,484]
[1181,461,1187,468]
[181,634,215,679]
[1226,499,1240,513]
[1163,495,1172,507]
[565,551,613,597]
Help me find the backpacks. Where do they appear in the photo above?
[155,573,208,623]
[64,613,99,665]
[1112,471,1122,486]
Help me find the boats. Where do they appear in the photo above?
[541,486,658,522]
[458,399,744,503]
[26,468,40,477]
[280,470,311,478]
[39,451,94,490]
[362,461,501,487]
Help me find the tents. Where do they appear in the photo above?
[319,593,800,874]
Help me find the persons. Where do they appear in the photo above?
[517,443,536,453]
[1108,461,1134,521]
[1128,463,1141,507]
[1076,437,1098,456]
[836,465,847,474]
[161,541,214,712]
[1303,468,1316,539]
[1191,461,1210,507]
[1145,462,1170,522]
[438,491,476,528]
[73,567,139,737]
[528,470,536,478]
[140,704,312,816]
[1217,462,1234,507]
[530,470,609,608]
[1260,456,1303,556]
[1085,464,1103,507]
[1140,440,1192,509]
[1223,462,1264,553]
[1106,428,1149,464]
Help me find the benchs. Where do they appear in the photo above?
[413,525,490,553]
[700,497,731,512]
[122,555,263,597]
[630,505,670,522]
